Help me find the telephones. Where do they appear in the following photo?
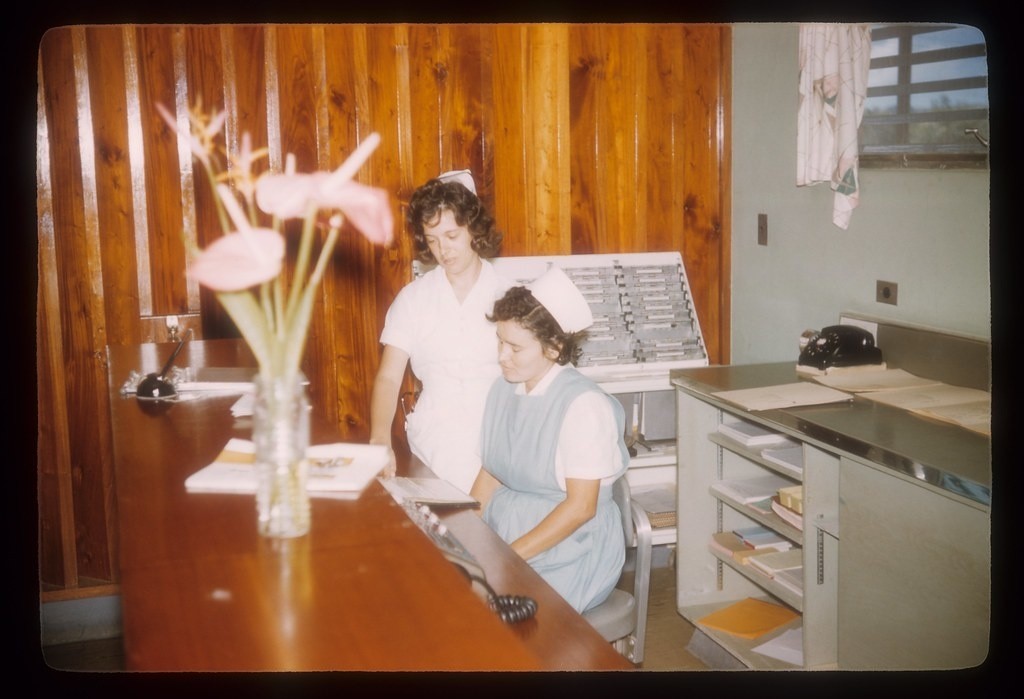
[798,324,882,370]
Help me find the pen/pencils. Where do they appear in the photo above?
[157,335,189,381]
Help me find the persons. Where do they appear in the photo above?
[370,169,521,494]
[470,271,628,617]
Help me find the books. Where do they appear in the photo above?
[625,421,804,639]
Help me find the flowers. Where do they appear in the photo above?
[149,96,397,378]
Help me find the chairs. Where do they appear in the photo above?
[580,473,652,666]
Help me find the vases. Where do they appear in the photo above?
[253,378,305,538]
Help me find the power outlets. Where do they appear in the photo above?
[144,316,225,342]
[877,280,896,305]
[757,214,767,246]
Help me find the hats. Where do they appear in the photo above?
[522,266,593,333]
[438,170,477,197]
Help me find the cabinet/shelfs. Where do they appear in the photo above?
[666,361,991,670]
[414,252,709,574]
